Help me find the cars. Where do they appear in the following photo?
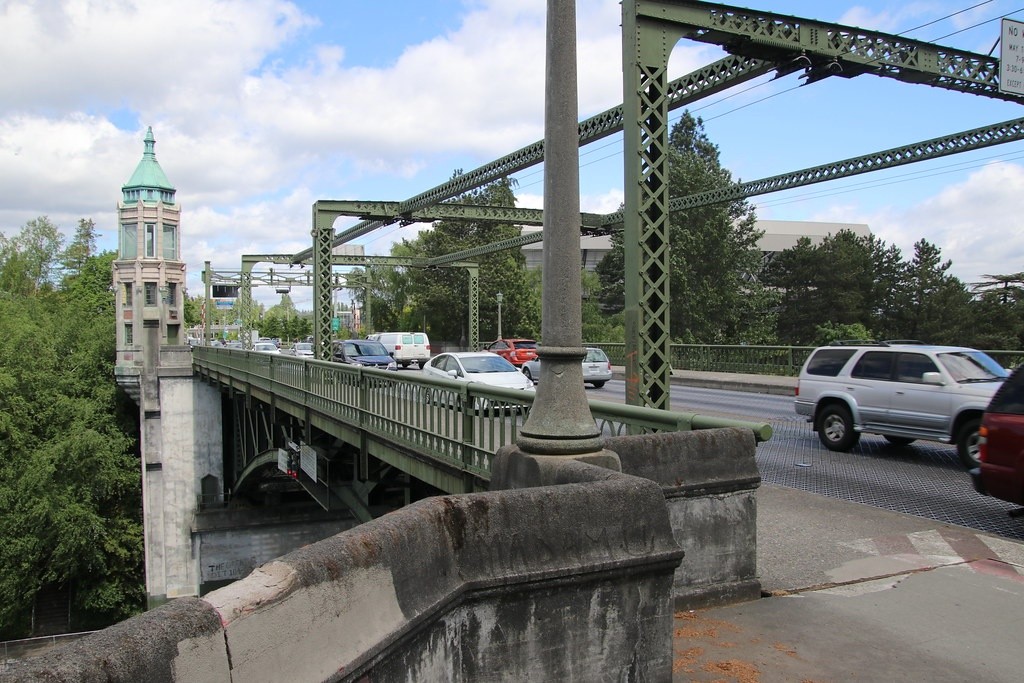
[481,338,539,368]
[968,355,1024,505]
[420,349,537,415]
[521,348,612,390]
[211,338,315,364]
[330,339,398,388]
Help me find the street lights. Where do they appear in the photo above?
[496,290,503,341]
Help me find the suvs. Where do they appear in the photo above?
[794,338,1013,471]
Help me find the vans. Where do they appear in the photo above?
[368,331,431,370]
